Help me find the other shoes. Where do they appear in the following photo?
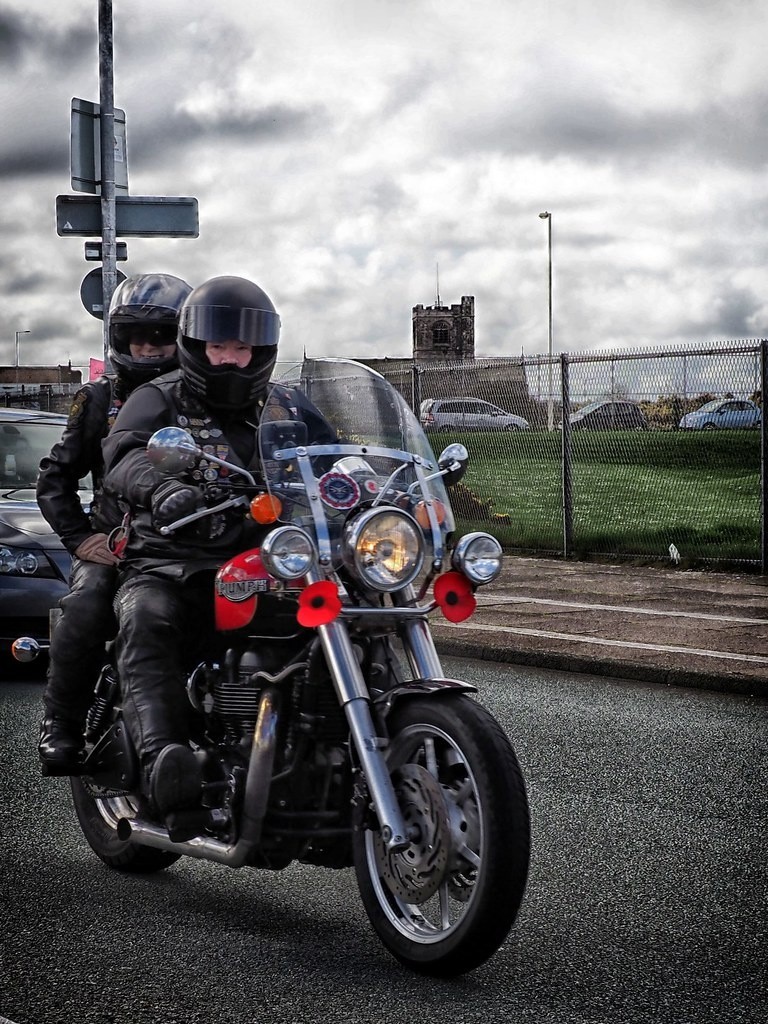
[139,740,199,827]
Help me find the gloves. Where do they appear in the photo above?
[74,530,122,567]
[150,481,204,523]
[350,468,409,504]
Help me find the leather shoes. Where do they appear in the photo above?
[35,711,85,764]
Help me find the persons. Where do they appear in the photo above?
[102,276,351,840]
[36,273,194,767]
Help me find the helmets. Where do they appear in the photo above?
[105,273,193,389]
[176,276,281,407]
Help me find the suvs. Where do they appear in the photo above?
[419,396,530,433]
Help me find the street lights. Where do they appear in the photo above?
[539,211,555,431]
[15,330,31,390]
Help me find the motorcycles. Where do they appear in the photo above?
[12,356,532,981]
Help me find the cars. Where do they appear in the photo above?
[557,401,648,431]
[0,408,95,649]
[679,398,761,430]
